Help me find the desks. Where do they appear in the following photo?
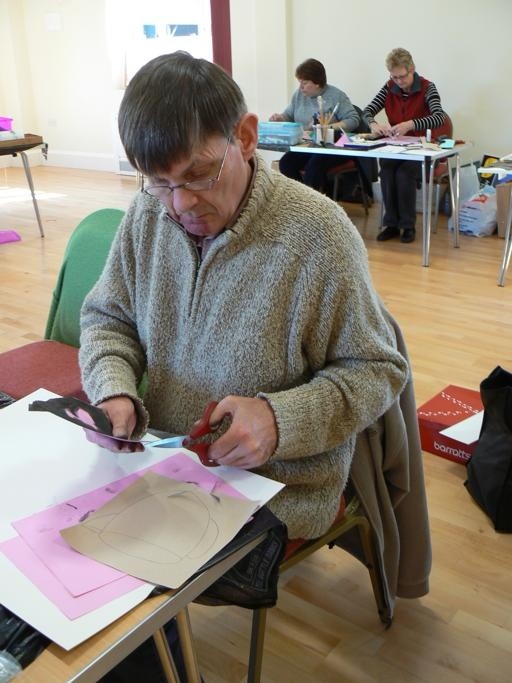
[0,133,47,242]
[477,155,512,287]
[256,129,476,269]
[0,393,272,682]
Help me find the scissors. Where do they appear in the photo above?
[135,401,223,470]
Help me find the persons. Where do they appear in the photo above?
[360,48,446,242]
[76,49,410,683]
[360,47,439,199]
[269,57,360,191]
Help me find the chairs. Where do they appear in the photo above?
[248,481,387,683]
[377,111,452,248]
[0,206,129,401]
[298,157,370,219]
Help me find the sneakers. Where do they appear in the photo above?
[377,226,399,242]
[400,225,417,244]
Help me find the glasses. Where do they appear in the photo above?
[386,70,415,84]
[132,133,235,196]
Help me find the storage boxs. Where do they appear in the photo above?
[414,383,485,465]
[495,180,512,238]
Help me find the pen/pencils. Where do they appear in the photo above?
[315,96,340,144]
